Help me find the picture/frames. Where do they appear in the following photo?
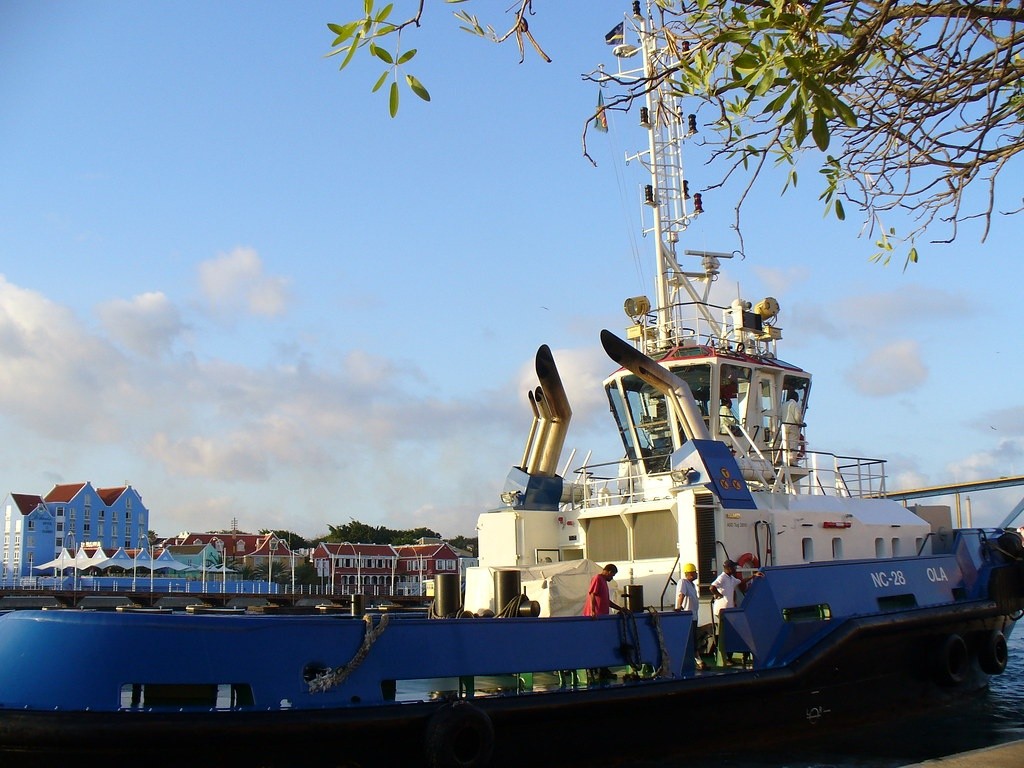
[534,548,560,564]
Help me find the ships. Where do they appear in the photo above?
[0,524,1024,768]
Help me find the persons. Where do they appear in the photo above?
[675,563,699,654]
[719,397,739,435]
[709,560,765,666]
[780,391,807,467]
[582,564,622,678]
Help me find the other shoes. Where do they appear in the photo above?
[727,659,736,665]
[599,670,617,679]
[696,663,711,671]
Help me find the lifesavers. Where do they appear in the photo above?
[797,433,805,461]
[736,552,760,592]
[937,633,971,685]
[978,629,1008,675]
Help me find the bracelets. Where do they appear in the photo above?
[752,573,755,577]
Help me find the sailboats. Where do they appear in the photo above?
[461,0,935,659]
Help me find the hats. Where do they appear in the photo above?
[723,559,739,567]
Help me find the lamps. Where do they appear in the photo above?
[816,602,832,621]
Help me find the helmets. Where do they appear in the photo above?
[683,563,697,572]
